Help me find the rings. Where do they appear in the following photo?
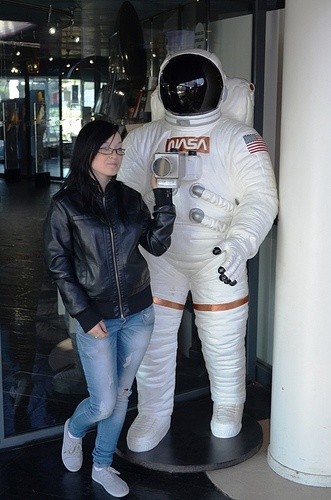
[95,334,98,338]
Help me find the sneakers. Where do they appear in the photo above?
[62,419,84,472]
[91,465,130,497]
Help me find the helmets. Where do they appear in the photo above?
[157,48,228,125]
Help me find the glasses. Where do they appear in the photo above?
[98,146,125,155]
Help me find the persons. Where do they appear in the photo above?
[34,91,45,166]
[37,116,178,498]
[111,46,282,451]
